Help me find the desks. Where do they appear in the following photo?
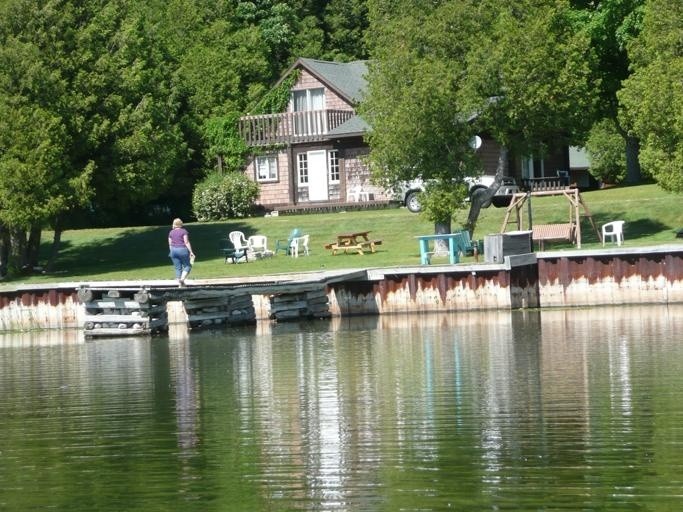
[223,249,249,264]
[332,229,371,256]
[413,233,463,265]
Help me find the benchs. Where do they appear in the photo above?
[332,246,362,249]
[326,243,337,249]
[344,240,364,244]
[360,240,382,246]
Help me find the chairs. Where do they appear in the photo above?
[275,228,300,256]
[229,231,267,261]
[557,170,570,186]
[602,220,625,247]
[290,234,309,258]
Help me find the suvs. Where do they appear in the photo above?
[385,156,516,213]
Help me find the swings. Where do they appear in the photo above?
[519,194,574,242]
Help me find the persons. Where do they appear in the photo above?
[167,218,196,288]
